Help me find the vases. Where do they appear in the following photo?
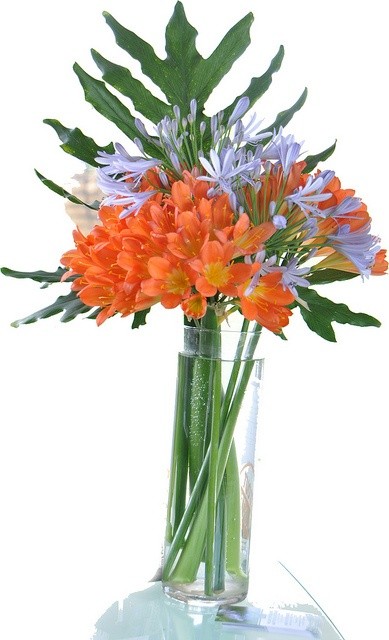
[156,321,267,610]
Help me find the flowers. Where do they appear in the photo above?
[0,2,389,601]
